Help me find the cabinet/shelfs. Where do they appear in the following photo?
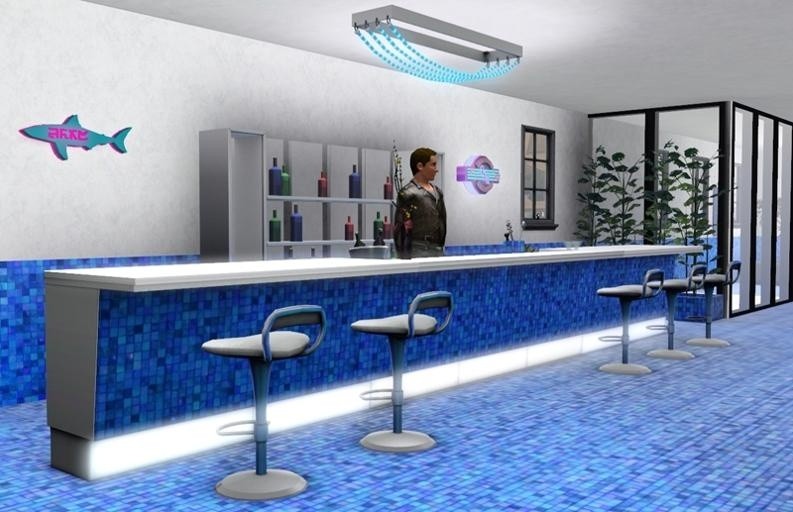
[199,129,412,260]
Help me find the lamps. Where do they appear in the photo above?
[351,5,522,84]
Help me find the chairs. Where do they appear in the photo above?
[201,304,327,500]
[351,291,453,454]
[645,264,708,360]
[596,268,665,375]
[686,260,741,347]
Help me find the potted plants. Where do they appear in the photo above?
[663,148,738,323]
